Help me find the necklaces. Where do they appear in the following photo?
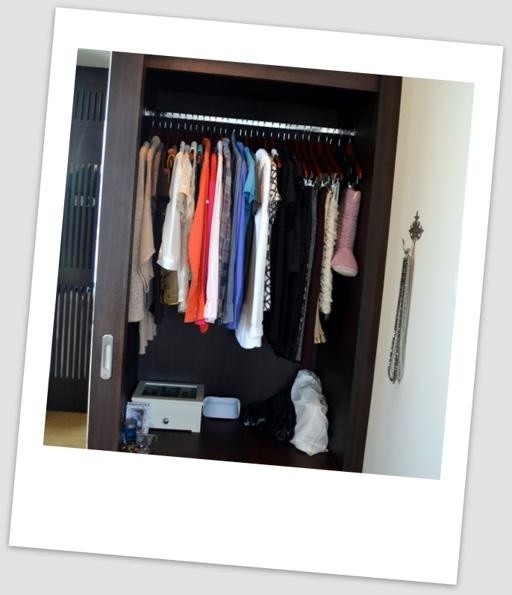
[387,258,409,385]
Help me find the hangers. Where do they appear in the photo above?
[138,107,362,187]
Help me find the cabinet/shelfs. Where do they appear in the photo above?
[88,52,402,472]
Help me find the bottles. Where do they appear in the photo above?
[124,418,137,442]
